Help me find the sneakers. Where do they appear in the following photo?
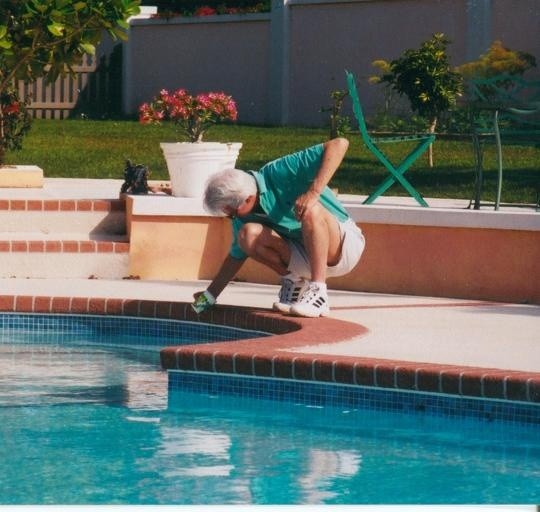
[273,278,310,315]
[290,286,329,318]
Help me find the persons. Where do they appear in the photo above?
[190,137,367,321]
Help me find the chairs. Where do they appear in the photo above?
[467,72,539,212]
[344,68,436,208]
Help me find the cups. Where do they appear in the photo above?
[190,289,217,314]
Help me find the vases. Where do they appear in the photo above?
[160,143,244,197]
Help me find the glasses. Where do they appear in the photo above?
[225,204,239,220]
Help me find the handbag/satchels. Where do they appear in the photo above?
[121,158,149,193]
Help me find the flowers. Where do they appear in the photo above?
[138,86,241,142]
